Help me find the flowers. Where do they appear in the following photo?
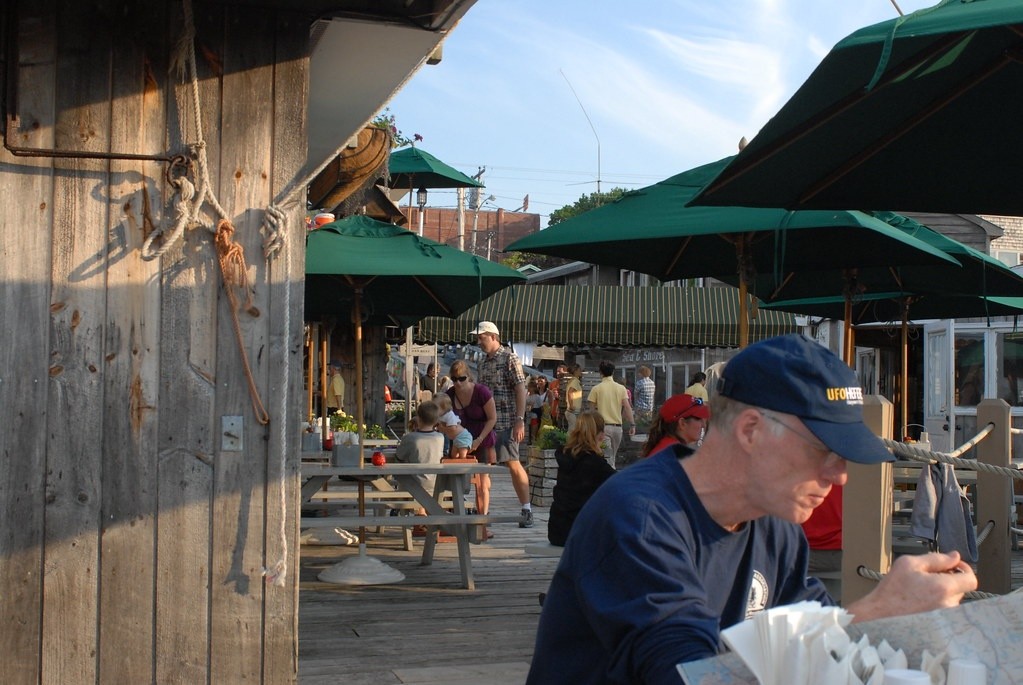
[385,404,417,421]
[330,410,389,440]
[526,424,609,451]
[372,108,423,147]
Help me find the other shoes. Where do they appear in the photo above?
[412,513,428,536]
[486,531,494,538]
[483,528,487,541]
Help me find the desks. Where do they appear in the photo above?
[301,462,525,590]
[892,469,977,525]
[893,457,1023,550]
[300,448,396,460]
[363,439,400,446]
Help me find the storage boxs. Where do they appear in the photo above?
[332,445,361,467]
[908,442,931,451]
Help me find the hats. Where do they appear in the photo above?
[660,394,710,421]
[716,333,896,465]
[327,359,342,368]
[469,321,500,335]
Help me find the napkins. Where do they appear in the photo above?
[333,431,360,445]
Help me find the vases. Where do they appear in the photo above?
[526,445,613,506]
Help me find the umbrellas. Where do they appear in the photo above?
[686,1,1023,217]
[306,215,523,556]
[501,153,1023,437]
[374,146,486,232]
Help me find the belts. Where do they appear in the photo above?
[605,424,622,427]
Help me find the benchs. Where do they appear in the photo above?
[310,489,461,498]
[302,511,529,529]
[302,498,474,511]
[892,489,978,552]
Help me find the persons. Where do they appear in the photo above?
[392,320,714,547]
[523,332,978,684]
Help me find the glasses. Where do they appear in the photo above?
[676,397,703,416]
[762,412,841,468]
[451,376,467,382]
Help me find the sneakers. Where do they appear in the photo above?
[519,508,535,528]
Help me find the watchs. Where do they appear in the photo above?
[516,416,524,421]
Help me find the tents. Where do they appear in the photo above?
[411,284,804,393]
